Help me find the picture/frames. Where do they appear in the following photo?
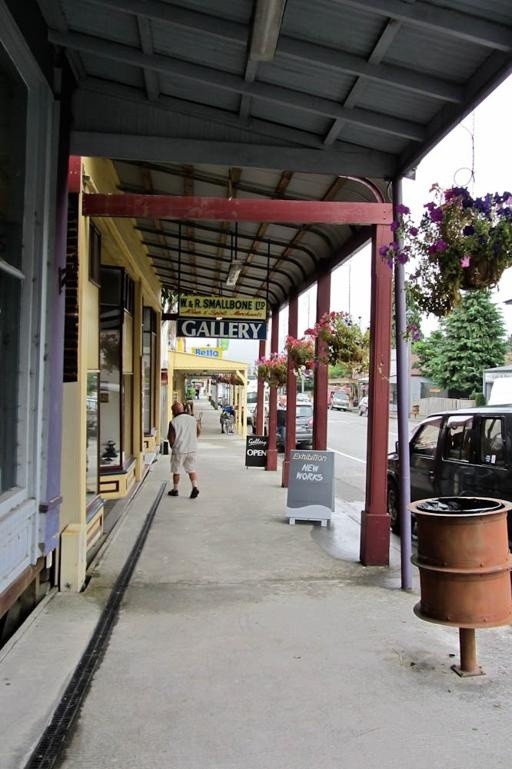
[88,217,102,289]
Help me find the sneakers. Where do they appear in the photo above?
[168,488,179,497]
[190,486,200,498]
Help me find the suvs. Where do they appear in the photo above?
[387,400,512,552]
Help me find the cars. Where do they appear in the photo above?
[356,395,368,417]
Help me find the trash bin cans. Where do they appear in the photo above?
[406,495,512,632]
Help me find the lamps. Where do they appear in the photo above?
[224,177,245,288]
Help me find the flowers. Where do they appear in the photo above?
[255,310,367,390]
[379,188,509,313]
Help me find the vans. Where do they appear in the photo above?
[217,381,353,449]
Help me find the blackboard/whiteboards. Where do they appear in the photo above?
[245,435,269,468]
[285,449,336,520]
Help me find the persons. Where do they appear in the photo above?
[168,401,202,499]
